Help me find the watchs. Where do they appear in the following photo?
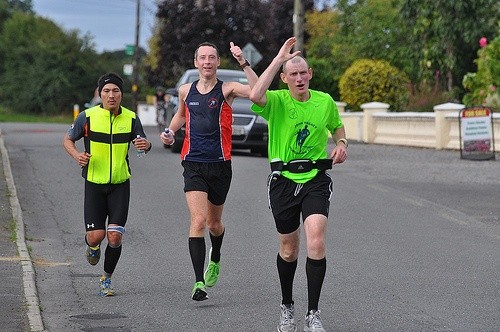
[240,59,250,70]
[337,138,348,148]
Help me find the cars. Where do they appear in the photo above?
[162,68,268,158]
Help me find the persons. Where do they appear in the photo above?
[63,73,152,297]
[250,37,349,332]
[160,42,259,301]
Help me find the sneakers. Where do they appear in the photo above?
[277,298,297,332]
[191,281,208,301]
[85,243,101,265]
[204,247,221,287]
[304,309,326,332]
[96,277,115,296]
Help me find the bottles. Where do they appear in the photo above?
[135,134,145,159]
[163,128,175,146]
